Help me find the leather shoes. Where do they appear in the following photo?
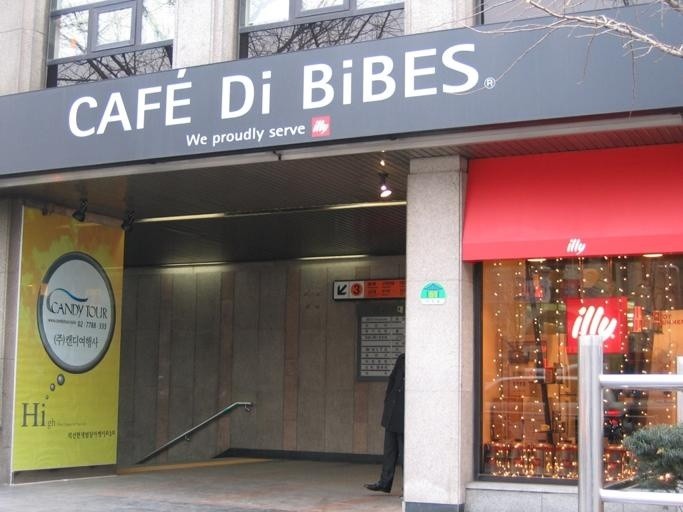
[363,483,390,492]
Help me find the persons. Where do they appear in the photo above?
[363,352,404,493]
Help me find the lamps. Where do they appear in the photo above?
[72,197,88,222]
[121,209,137,232]
[378,170,392,198]
